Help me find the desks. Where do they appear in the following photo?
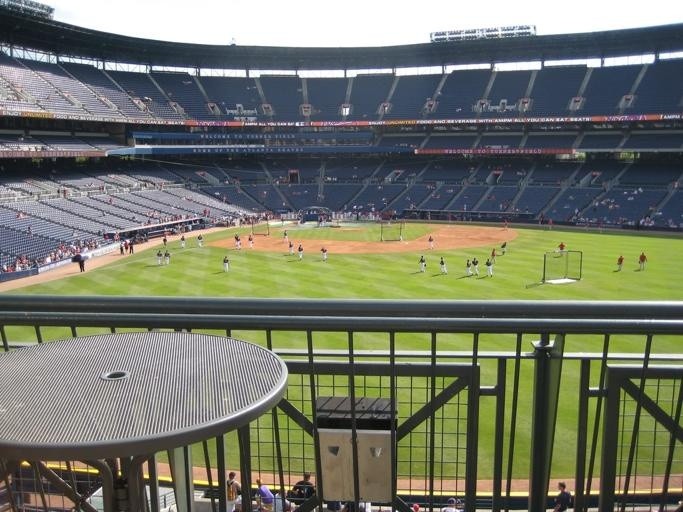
[1,332,289,512]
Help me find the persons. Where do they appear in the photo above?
[466,259,473,276]
[269,487,298,512]
[289,472,314,506]
[440,498,460,512]
[550,481,571,512]
[405,182,682,230]
[472,257,478,275]
[558,242,566,256]
[418,255,426,272]
[0,182,400,280]
[617,255,624,272]
[428,233,434,250]
[484,258,492,277]
[252,478,274,512]
[501,242,506,255]
[440,256,448,275]
[490,248,496,264]
[639,252,647,272]
[225,472,241,512]
